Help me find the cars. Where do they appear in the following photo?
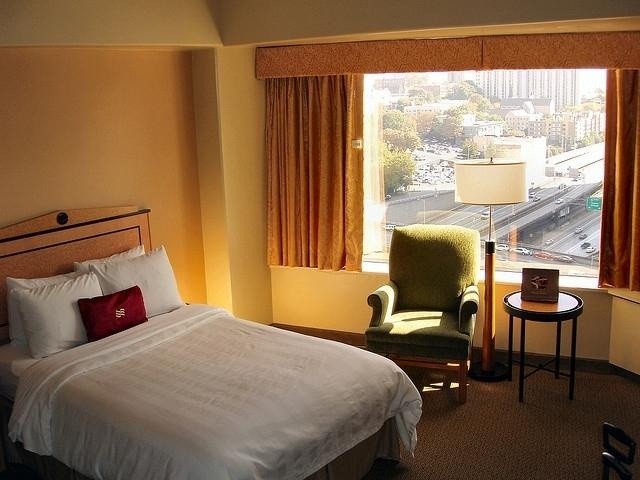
[529,192,541,202]
[555,197,564,204]
[575,228,596,253]
[496,244,574,263]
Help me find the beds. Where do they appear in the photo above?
[2,206,422,480]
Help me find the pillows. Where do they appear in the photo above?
[2,244,187,358]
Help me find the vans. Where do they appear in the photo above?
[481,211,493,220]
[382,225,396,231]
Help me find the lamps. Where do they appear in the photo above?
[456,159,526,381]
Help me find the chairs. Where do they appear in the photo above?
[365,224,479,405]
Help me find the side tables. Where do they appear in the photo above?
[501,290,582,403]
